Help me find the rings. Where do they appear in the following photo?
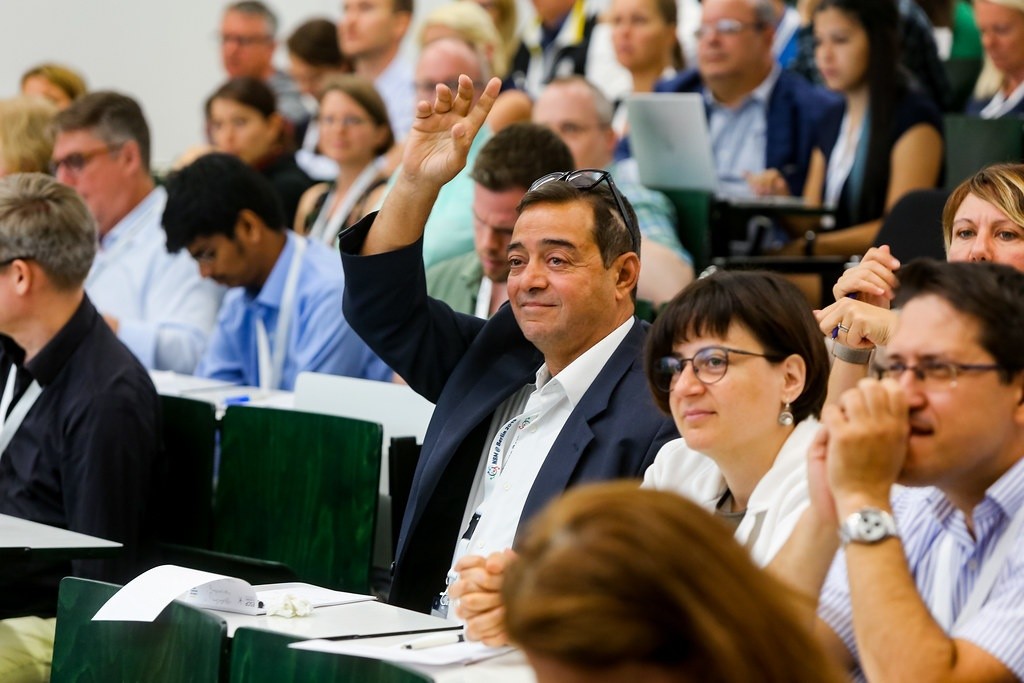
[837,322,849,332]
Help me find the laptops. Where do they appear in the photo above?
[623,91,803,207]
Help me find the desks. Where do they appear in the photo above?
[200,580,534,683]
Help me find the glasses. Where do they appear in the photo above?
[874,352,1003,390]
[224,34,270,45]
[44,144,126,177]
[653,346,786,393]
[694,19,764,39]
[319,114,365,128]
[526,168,638,253]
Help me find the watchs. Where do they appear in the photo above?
[838,507,900,544]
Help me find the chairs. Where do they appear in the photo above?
[50,391,438,683]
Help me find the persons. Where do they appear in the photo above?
[51,91,231,375]
[0,173,170,622]
[161,0,1024,683]
[21,62,91,112]
[0,98,58,175]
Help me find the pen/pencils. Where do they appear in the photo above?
[831,292,857,340]
[221,396,249,403]
[403,634,464,649]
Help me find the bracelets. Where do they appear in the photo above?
[831,339,871,364]
[802,231,817,256]
